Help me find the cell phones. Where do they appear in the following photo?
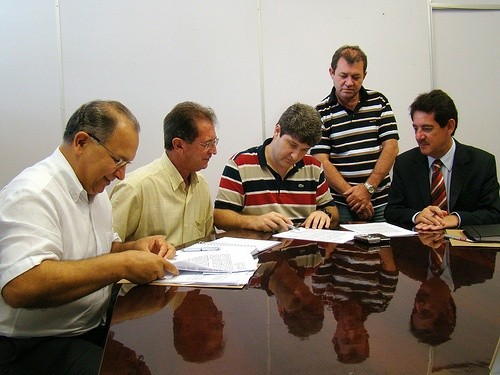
[354,234,380,244]
[368,234,390,243]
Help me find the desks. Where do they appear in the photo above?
[97,218,500,375]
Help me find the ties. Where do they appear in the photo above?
[431,160,448,214]
[432,243,446,275]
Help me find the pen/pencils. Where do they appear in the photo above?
[287,224,300,231]
[443,234,474,243]
[430,212,436,217]
[183,247,220,251]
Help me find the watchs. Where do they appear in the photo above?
[364,182,376,194]
[321,208,332,218]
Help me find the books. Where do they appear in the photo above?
[462,225,500,243]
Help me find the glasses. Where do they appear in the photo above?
[88,133,129,168]
[191,138,219,149]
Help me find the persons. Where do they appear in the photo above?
[99,330,151,375]
[214,104,339,232]
[226,233,323,336]
[110,102,214,247]
[0,100,178,375]
[122,284,224,363]
[310,46,399,222]
[312,234,398,364]
[390,230,497,346]
[383,91,500,230]
